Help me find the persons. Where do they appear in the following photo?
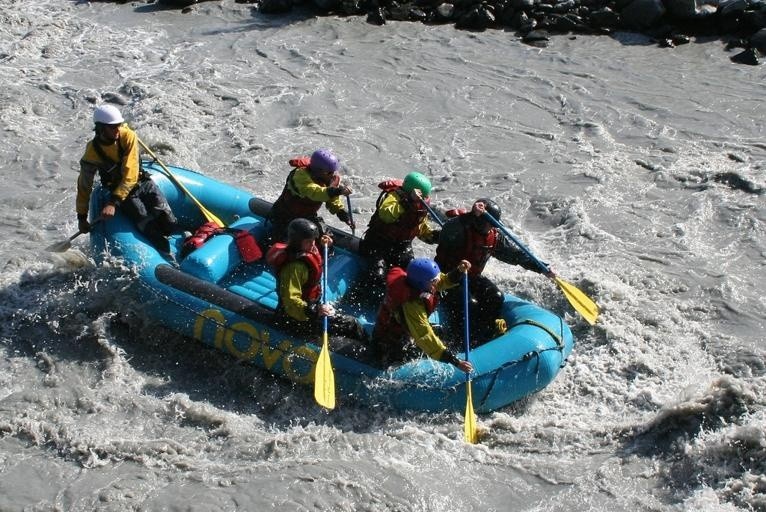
[76,105,179,269]
[358,173,442,309]
[434,199,556,347]
[276,217,367,342]
[369,256,473,372]
[266,149,356,255]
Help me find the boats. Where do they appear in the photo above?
[86,156,578,421]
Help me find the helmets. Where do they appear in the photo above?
[470,196,506,229]
[92,105,125,125]
[286,217,322,248]
[400,170,433,200]
[309,147,342,173]
[404,257,443,289]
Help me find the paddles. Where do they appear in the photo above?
[459,262,481,446]
[481,206,600,327]
[136,142,227,232]
[312,240,337,412]
